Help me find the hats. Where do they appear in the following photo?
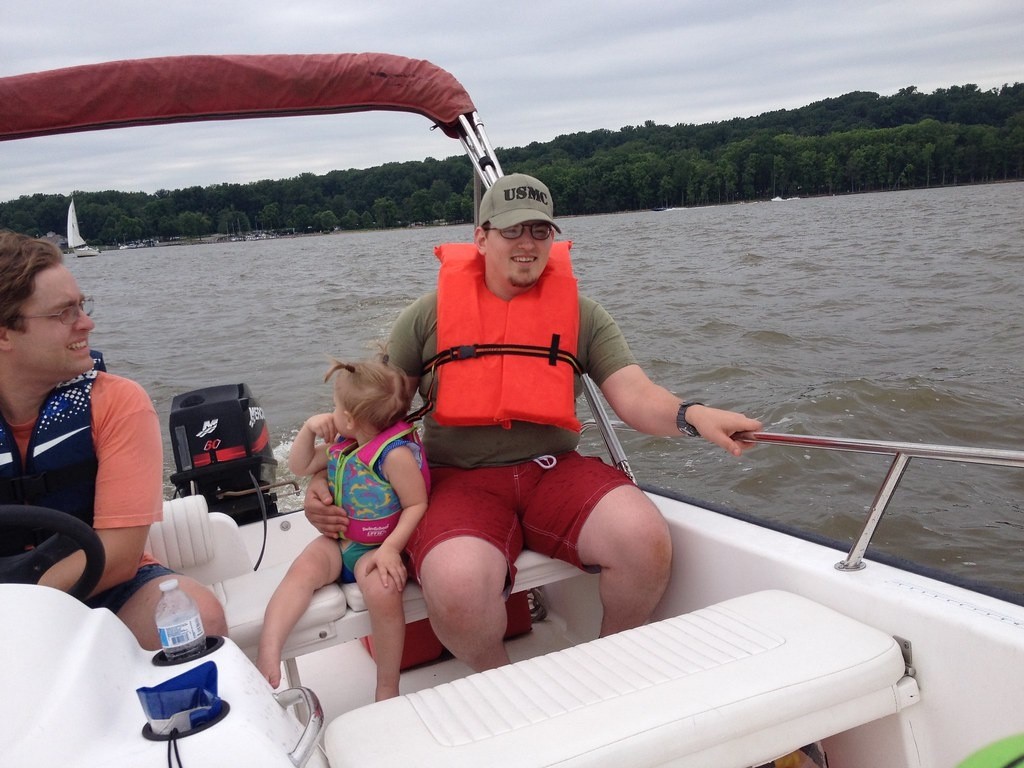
[478,172,562,234]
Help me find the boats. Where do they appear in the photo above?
[0,50,1024,768]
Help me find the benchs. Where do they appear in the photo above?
[139,493,595,731]
[322,588,921,768]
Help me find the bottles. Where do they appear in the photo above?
[154,579,207,661]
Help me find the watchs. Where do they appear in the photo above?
[676,400,707,439]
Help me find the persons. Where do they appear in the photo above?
[255,339,429,704]
[301,170,768,677]
[1,228,232,656]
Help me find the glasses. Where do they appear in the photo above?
[10,296,95,325]
[484,223,552,241]
